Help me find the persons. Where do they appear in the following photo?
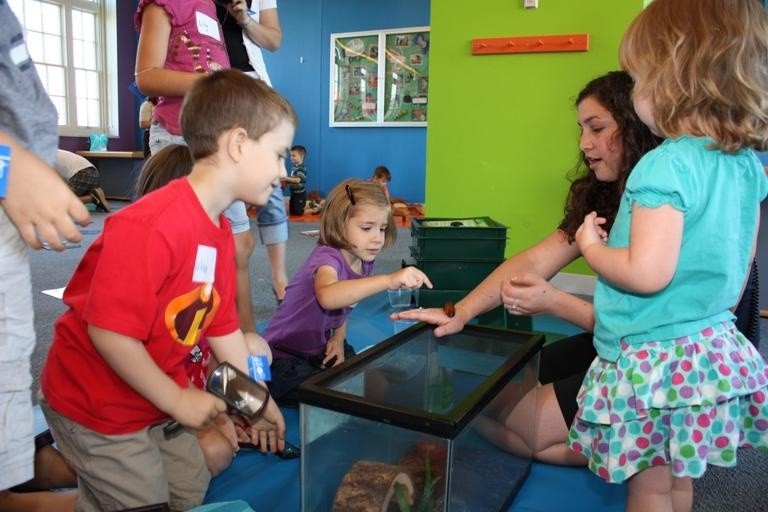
[282,144,326,218]
[132,1,256,335]
[366,164,391,206]
[0,69,298,510]
[136,94,158,158]
[211,1,291,305]
[385,67,762,470]
[54,146,111,213]
[259,176,435,409]
[130,144,276,479]
[0,1,93,491]
[562,1,768,510]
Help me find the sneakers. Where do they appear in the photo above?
[90,188,111,213]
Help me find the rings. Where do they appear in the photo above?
[511,303,518,310]
[418,306,423,311]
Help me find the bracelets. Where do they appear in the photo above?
[238,16,252,29]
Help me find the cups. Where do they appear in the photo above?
[387,287,412,307]
